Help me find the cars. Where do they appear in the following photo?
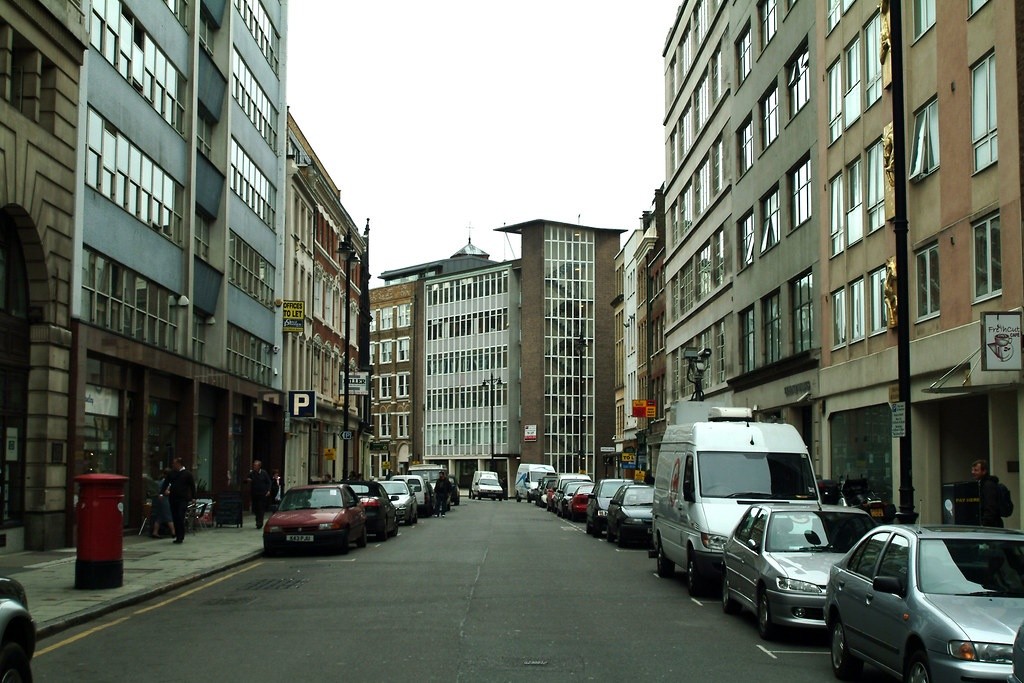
[823,524,1024,683]
[263,484,368,558]
[606,485,654,548]
[448,473,460,506]
[721,504,879,641]
[0,576,37,683]
[585,479,646,538]
[534,473,592,513]
[378,481,417,526]
[557,482,596,519]
[335,481,400,543]
[390,475,434,518]
[566,485,594,522]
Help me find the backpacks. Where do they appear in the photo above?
[998,482,1014,517]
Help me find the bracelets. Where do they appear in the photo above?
[159,494,163,496]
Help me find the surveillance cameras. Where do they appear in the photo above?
[797,392,812,402]
[698,348,712,358]
[682,346,699,359]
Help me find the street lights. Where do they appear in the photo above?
[575,333,589,470]
[335,227,361,480]
[481,373,503,473]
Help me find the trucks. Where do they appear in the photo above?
[472,471,503,502]
[408,464,451,511]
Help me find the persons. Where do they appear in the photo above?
[151,467,175,539]
[324,475,335,482]
[247,460,273,529]
[971,460,1004,528]
[159,457,196,544]
[269,469,285,514]
[340,471,364,481]
[644,470,655,485]
[433,471,452,518]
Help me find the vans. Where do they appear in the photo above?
[515,463,557,504]
[651,407,823,597]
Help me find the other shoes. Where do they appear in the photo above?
[152,535,160,538]
[441,514,445,517]
[257,524,263,529]
[171,534,176,538]
[173,539,183,543]
[434,515,437,517]
